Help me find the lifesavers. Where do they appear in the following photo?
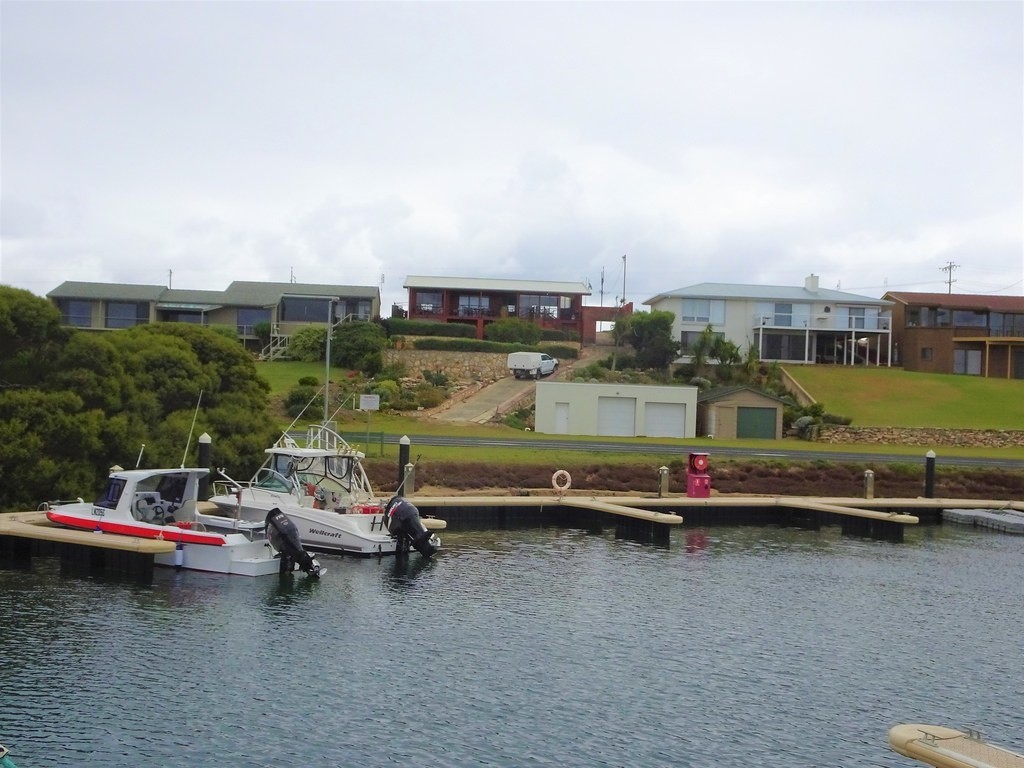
[552,469,573,491]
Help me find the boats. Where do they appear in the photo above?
[45,388,328,581]
[207,387,443,558]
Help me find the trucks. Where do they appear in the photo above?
[506,352,559,380]
